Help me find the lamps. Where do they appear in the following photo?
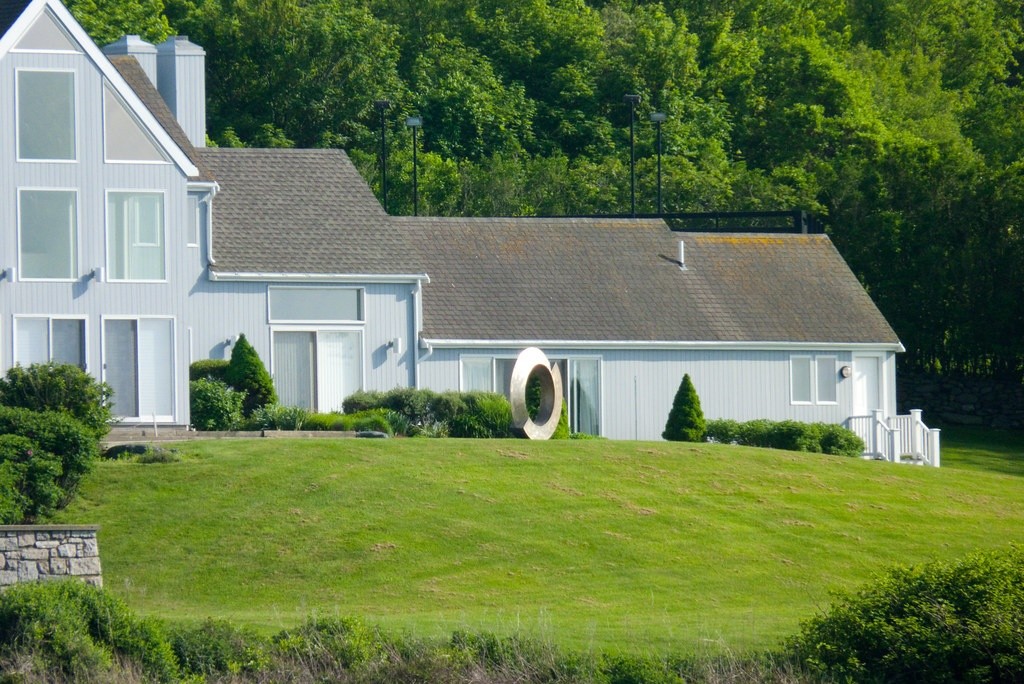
[840,366,851,378]
[91,266,104,282]
[2,267,15,282]
[226,335,239,351]
[389,338,401,353]
